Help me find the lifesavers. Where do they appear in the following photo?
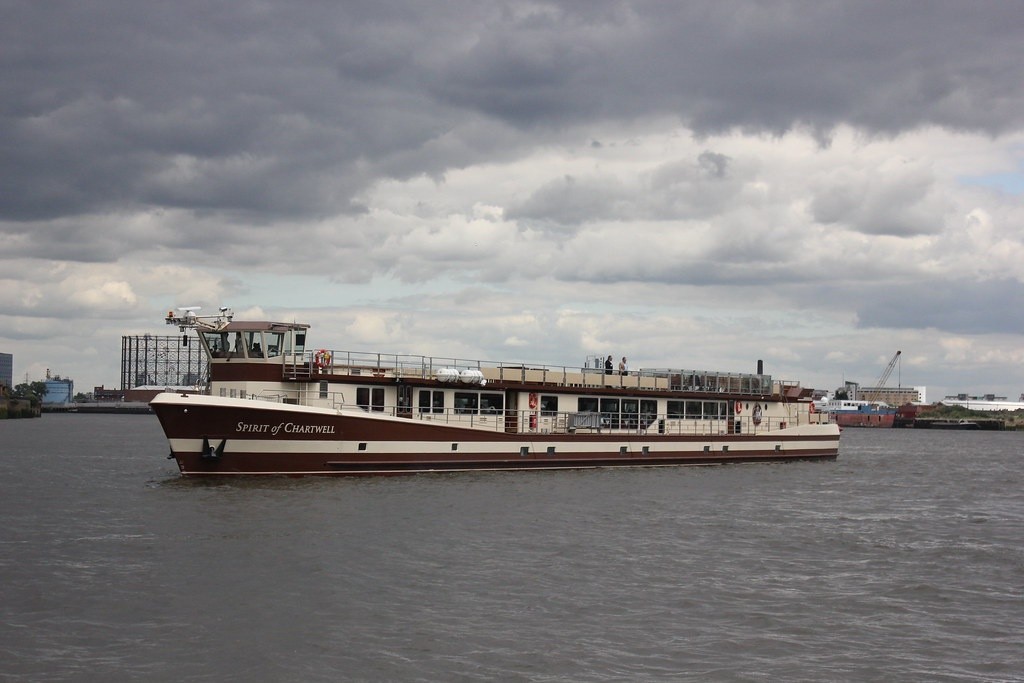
[809,402,816,414]
[736,400,742,413]
[315,347,331,368]
[528,393,539,408]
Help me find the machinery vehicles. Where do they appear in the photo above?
[867,351,901,409]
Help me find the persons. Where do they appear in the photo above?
[217,332,247,353]
[604,354,628,376]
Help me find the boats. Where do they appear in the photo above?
[812,396,895,428]
[931,418,976,430]
[149,306,841,480]
